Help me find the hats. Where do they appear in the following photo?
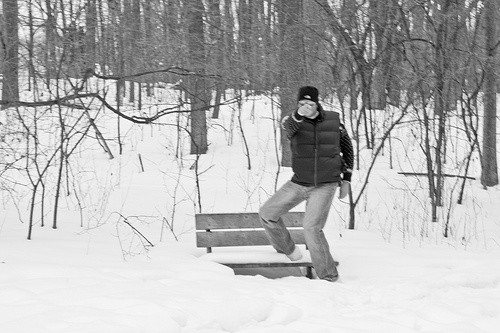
[296,86,319,103]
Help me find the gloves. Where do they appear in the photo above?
[298,104,316,117]
[338,182,349,199]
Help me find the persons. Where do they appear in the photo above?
[257,85,354,282]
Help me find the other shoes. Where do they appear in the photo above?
[287,246,303,261]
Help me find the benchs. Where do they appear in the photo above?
[195,212,339,278]
